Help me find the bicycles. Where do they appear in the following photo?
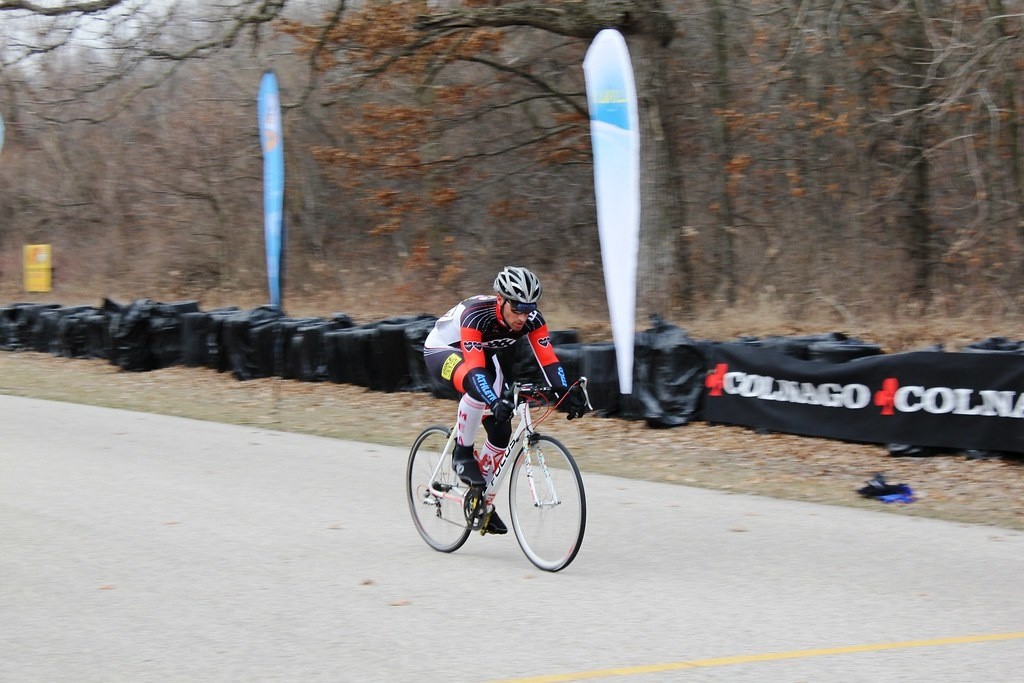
[406,375,590,574]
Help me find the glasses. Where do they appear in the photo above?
[500,294,533,314]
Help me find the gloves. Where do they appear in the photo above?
[490,398,514,425]
[558,386,586,420]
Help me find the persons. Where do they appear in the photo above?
[423,266,585,534]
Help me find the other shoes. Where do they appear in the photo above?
[480,506,507,534]
[452,437,486,487]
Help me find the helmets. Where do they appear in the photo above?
[493,266,543,303]
